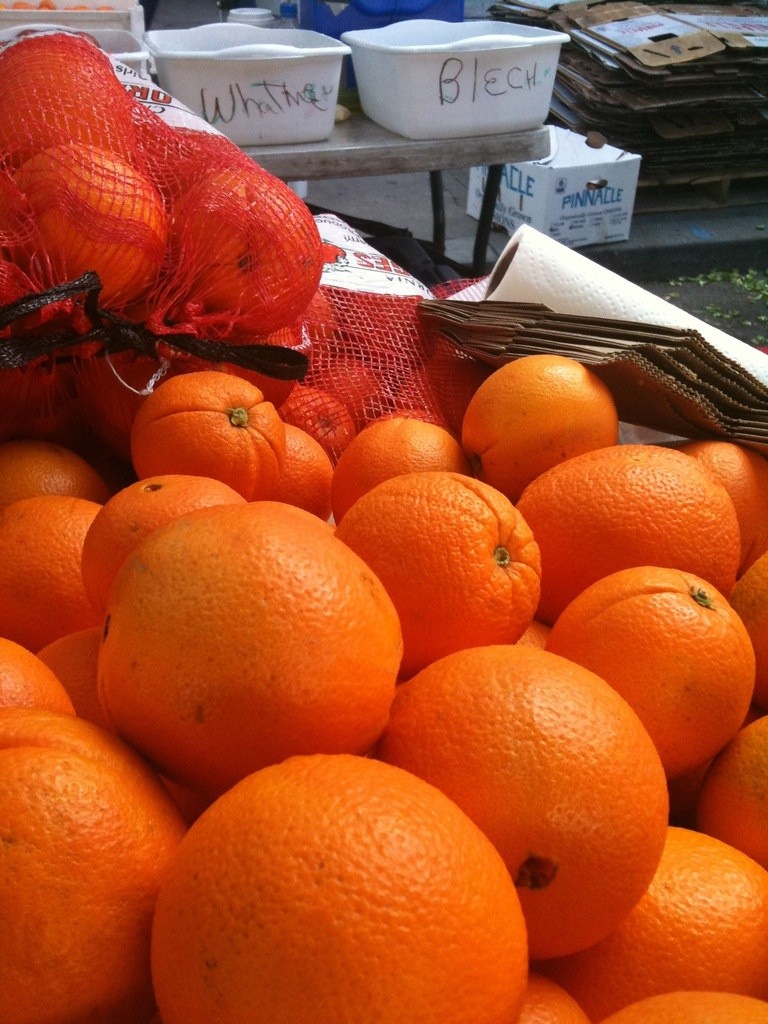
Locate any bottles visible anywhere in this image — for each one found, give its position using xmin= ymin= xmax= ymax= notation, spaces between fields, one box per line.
xmin=226 ymin=7 xmax=276 ymax=29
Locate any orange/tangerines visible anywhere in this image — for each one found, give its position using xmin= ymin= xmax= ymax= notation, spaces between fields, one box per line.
xmin=0 ymin=31 xmax=768 ymax=1024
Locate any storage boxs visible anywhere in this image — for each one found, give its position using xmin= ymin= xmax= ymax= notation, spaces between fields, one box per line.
xmin=467 ymin=123 xmax=642 ymax=251
xmin=0 ymin=0 xmax=352 ymax=148
xmin=342 ymin=19 xmax=571 ymax=139
